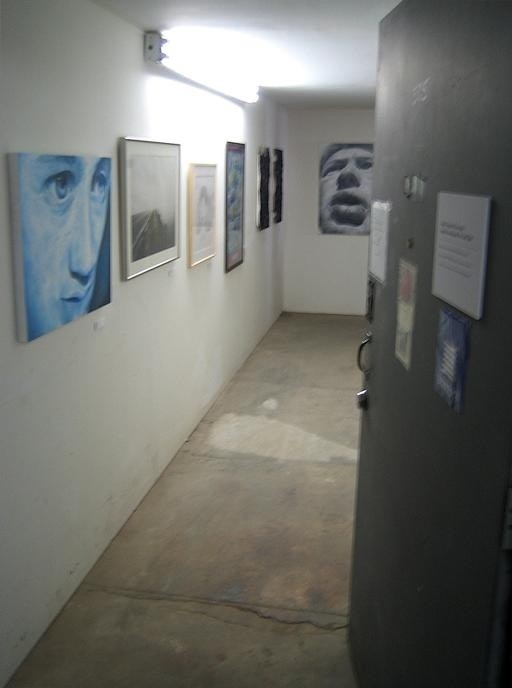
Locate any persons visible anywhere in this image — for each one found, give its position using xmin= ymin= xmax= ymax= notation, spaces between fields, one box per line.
xmin=19 ymin=151 xmax=110 ymax=341
xmin=317 ymin=143 xmax=373 ymax=235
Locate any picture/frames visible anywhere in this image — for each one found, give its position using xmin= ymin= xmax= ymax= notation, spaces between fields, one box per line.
xmin=118 ymin=136 xmax=246 ymax=281
